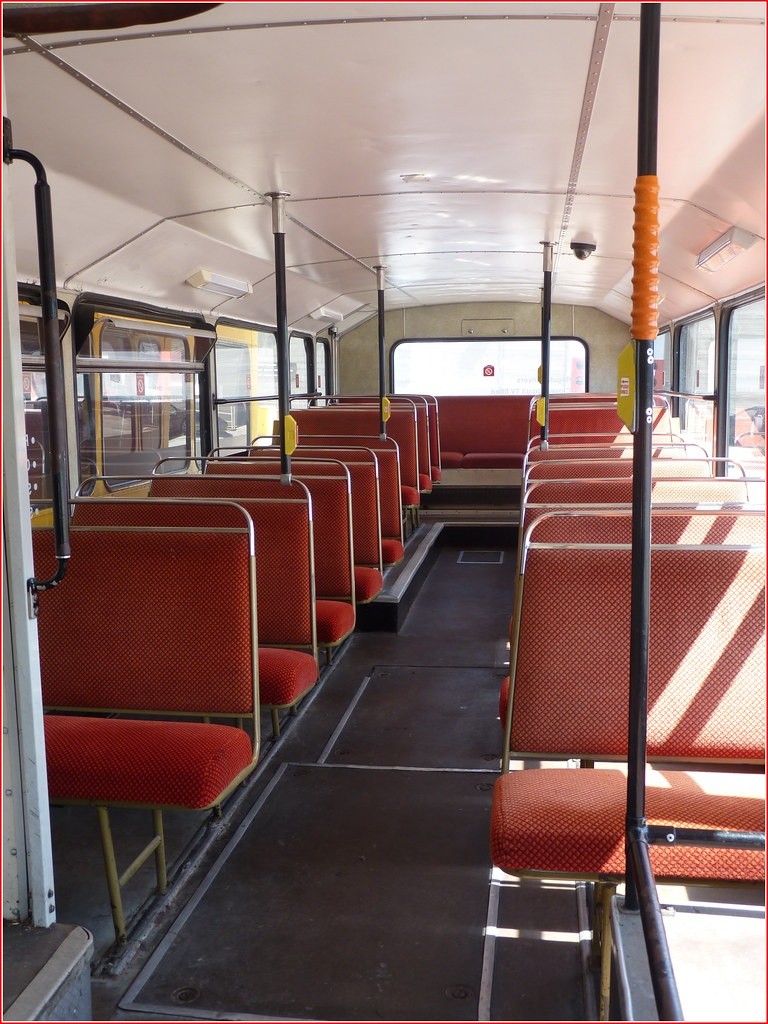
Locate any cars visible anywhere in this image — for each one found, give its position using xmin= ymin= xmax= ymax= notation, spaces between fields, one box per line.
xmin=705 ymin=405 xmax=766 ymax=448
xmin=143 ymin=403 xmax=188 ymax=437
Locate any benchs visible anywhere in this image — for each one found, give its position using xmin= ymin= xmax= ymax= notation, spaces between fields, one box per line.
xmin=335 ymin=394 xmax=672 ymax=473
xmin=23 ymin=390 xmax=250 ymax=514
xmin=70 ymin=474 xmax=319 ymax=821
xmin=508 ymin=456 xmax=749 ymax=650
xmin=30 ymin=496 xmax=263 ymax=948
xmin=150 ymin=455 xmax=358 ymax=719
xmin=203 ymin=445 xmax=385 ymax=668
xmin=246 ymin=395 xmax=442 ymax=569
xmin=683 ymin=396 xmax=766 ymax=478
xmin=490 ymin=508 xmax=766 ymax=1024
xmin=520 ymin=393 xmax=714 ymax=489
xmin=498 ymin=477 xmax=768 ymax=769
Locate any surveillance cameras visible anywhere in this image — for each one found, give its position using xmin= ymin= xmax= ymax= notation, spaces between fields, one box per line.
xmin=570 ymin=240 xmax=597 ymax=260
